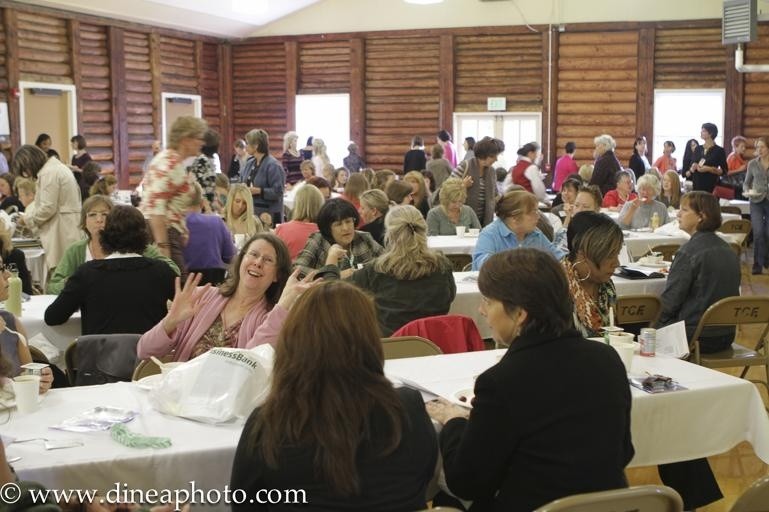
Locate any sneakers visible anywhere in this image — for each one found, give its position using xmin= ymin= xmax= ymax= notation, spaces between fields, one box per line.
xmin=751 ymin=263 xmax=762 ymax=273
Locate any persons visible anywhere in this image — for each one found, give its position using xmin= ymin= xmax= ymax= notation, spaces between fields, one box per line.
xmin=48 ymin=194 xmax=182 ymax=297
xmin=424 ymin=249 xmax=632 ymax=512
xmin=232 ymin=280 xmax=439 ymax=512
xmin=1 ymin=130 xmax=119 ymax=276
xmin=46 ymin=205 xmax=177 ymax=335
xmin=130 ymin=115 xmax=769 ymax=376
xmin=1 ymin=211 xmax=35 ymax=294
xmin=1 ymin=256 xmax=55 ymax=395
xmin=1 ymin=440 xmax=64 ymax=512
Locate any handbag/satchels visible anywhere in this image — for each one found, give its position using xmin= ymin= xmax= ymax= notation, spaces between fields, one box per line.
xmin=717 ymin=170 xmax=745 ymax=188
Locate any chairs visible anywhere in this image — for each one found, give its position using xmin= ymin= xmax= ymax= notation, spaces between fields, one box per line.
xmin=0 ymin=183 xmax=768 ymax=512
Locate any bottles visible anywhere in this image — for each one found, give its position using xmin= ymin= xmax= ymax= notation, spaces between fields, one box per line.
xmin=651 ymin=212 xmax=660 ymax=229
xmin=5 ymin=268 xmax=23 ymax=319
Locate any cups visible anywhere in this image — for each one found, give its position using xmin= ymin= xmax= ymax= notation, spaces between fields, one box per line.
xmin=648 ymin=254 xmax=663 ymax=264
xmin=468 ymin=228 xmax=480 ymax=235
xmin=10 ymin=375 xmax=41 ymax=416
xmin=20 ymin=363 xmax=49 ymax=378
xmin=749 ymin=189 xmax=757 ymax=194
xmin=456 ymin=225 xmax=466 ymax=238
xmin=601 ymin=326 xmax=625 ymax=343
xmin=607 ymin=332 xmax=636 ymax=372
xmin=234 ymin=234 xmax=245 ymax=246
xmin=160 ymin=362 xmax=182 ymax=378
xmin=119 ymin=190 xmax=132 ymax=203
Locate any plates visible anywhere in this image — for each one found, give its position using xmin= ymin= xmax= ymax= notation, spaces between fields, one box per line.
xmin=638 ymin=262 xmax=667 ymax=268
xmin=464 ymin=233 xmax=480 ymax=238
xmin=742 ymin=192 xmax=761 ymax=198
xmin=135 ymin=372 xmax=161 ymax=392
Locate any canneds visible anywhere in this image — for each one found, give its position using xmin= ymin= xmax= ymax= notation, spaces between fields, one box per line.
xmin=640 ymin=328 xmax=656 ymax=356
